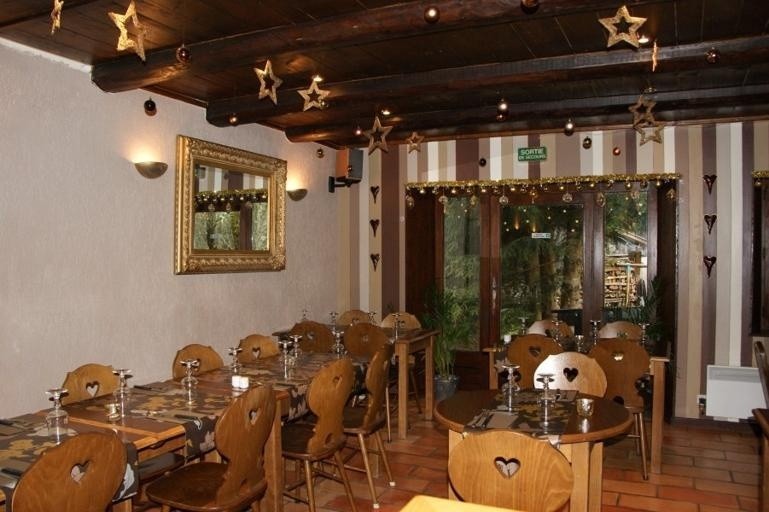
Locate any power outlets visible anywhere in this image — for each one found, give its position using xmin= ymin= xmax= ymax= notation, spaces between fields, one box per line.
xmin=696 ymin=394 xmax=707 ymax=406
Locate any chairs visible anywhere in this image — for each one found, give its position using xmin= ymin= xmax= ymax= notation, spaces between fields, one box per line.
xmin=280 ymin=359 xmax=358 ymax=512
xmin=289 ymin=319 xmax=338 ymax=357
xmin=237 ymin=334 xmax=281 ymax=366
xmin=506 ymin=333 xmax=565 ymax=389
xmin=145 ymin=385 xmax=277 ymax=512
xmin=343 ymin=322 xmax=412 ymax=443
xmin=587 ymin=338 xmax=650 ymax=481
xmin=400 ymin=495 xmax=530 ymax=512
xmin=526 ymin=318 xmax=575 ymax=346
xmin=302 ymin=343 xmax=396 ymax=509
xmin=173 ymin=343 xmax=225 ymax=381
xmin=533 ymin=352 xmax=607 ymax=398
xmin=59 ymin=363 xmax=129 ymax=407
xmin=381 ymin=311 xmax=424 ymax=415
xmin=449 ymin=431 xmax=573 ymax=512
xmin=597 ymin=320 xmax=650 ymax=456
xmin=753 ymin=341 xmax=769 ymax=409
xmin=10 ymin=432 xmax=128 ymax=512
xmin=336 ymin=308 xmax=373 ymax=335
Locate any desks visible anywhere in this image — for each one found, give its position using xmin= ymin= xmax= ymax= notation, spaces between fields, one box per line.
xmin=273 ymin=322 xmax=441 ymax=441
xmin=236 ymin=349 xmax=398 ymax=480
xmin=166 ymin=359 xmax=368 ymax=419
xmin=1 ymin=412 xmax=158 ymax=512
xmin=38 ymin=380 xmax=291 ymax=512
xmin=482 ymin=333 xmax=672 ymax=475
xmin=432 ymin=388 xmax=635 ymax=512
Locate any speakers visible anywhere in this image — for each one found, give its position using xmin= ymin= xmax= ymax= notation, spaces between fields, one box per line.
xmin=336 ymin=149 xmax=362 ymax=181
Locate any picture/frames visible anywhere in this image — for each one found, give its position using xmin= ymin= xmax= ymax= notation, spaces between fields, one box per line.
xmin=173 ymin=134 xmax=287 ymax=274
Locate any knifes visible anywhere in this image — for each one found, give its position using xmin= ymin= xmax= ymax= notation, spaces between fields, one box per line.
xmin=0 ymin=418 xmax=27 ymax=430
xmin=155 ymin=414 xmax=198 ymax=421
xmin=481 ymin=414 xmax=494 ymax=429
xmin=555 ymin=388 xmax=561 ymax=401
xmin=135 ymin=385 xmax=162 ymax=392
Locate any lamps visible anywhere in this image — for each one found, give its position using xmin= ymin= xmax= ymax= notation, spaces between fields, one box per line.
xmin=497 ymin=96 xmax=509 ymax=115
xmin=228 ymin=112 xmax=239 ymax=126
xmin=425 ymin=4 xmax=440 ymax=25
xmin=135 ymin=161 xmax=168 ymax=179
xmin=636 ymin=32 xmax=652 ymax=47
xmin=520 ymin=0 xmax=541 ymax=17
xmin=378 ymin=103 xmax=395 ymax=118
xmin=564 ymin=117 xmax=574 ymax=137
xmin=286 ymin=187 xmax=308 ymax=202
xmin=143 ymin=98 xmax=157 ymax=117
xmin=352 ymin=126 xmax=363 ymax=139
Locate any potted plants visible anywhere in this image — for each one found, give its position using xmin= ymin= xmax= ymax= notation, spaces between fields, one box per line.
xmin=600 ymin=275 xmax=673 ymax=358
xmin=421 ymin=284 xmax=480 ymax=400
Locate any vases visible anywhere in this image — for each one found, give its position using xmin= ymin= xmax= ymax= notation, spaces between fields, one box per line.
xmin=434 ymin=374 xmax=460 ymax=401
